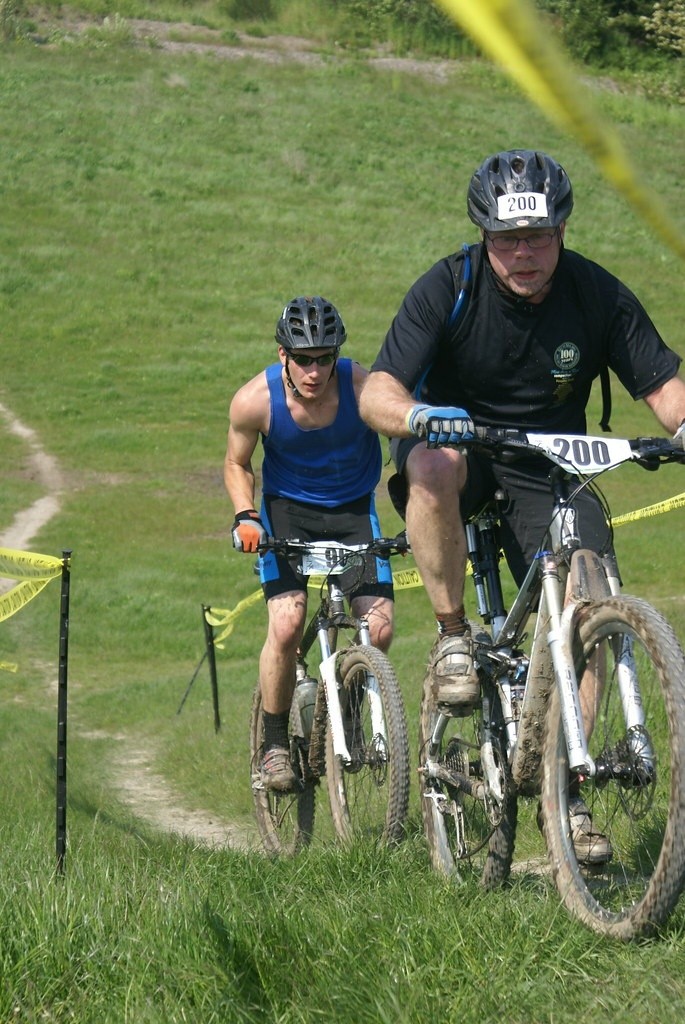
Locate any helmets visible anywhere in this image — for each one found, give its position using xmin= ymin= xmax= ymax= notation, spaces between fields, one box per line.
xmin=465 ymin=149 xmax=575 ymax=234
xmin=274 ymin=297 xmax=351 ymax=351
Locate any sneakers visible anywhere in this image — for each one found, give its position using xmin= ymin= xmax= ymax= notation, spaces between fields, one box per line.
xmin=258 ymin=743 xmax=301 ymax=795
xmin=429 ymin=628 xmax=480 ymax=706
xmin=536 ymin=791 xmax=614 ymax=862
xmin=342 ymin=706 xmax=367 ymax=774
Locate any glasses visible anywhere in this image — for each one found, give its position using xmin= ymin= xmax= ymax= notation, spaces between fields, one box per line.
xmin=485 ymin=229 xmax=559 ymax=251
xmin=287 ymin=349 xmax=337 ymax=367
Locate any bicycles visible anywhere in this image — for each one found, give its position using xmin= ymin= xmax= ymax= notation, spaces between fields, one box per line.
xmin=415 ymin=423 xmax=684 ymax=947
xmin=233 ymin=526 xmax=415 ymax=863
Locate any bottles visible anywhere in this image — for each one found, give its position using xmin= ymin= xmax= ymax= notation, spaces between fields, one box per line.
xmin=295 ymin=675 xmax=318 ymax=745
xmin=509 ymin=659 xmax=529 ymax=736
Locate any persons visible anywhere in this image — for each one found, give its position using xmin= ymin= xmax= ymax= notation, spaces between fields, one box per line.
xmin=358 ymin=137 xmax=683 ymax=862
xmin=223 ymin=297 xmax=399 ymax=795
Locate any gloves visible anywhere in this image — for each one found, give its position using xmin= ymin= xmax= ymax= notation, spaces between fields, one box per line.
xmin=672 ymin=418 xmax=685 ymax=452
xmin=229 ymin=508 xmax=269 ymax=556
xmin=404 ymin=404 xmax=476 ymax=456
